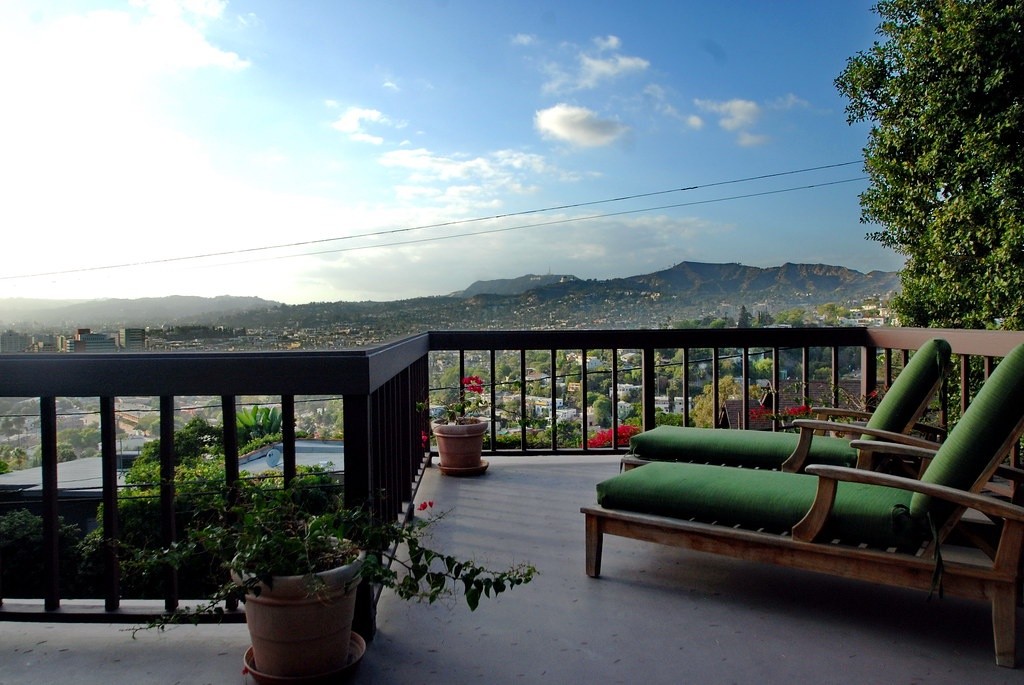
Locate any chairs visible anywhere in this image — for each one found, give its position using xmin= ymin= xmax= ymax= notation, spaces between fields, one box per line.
xmin=580 ymin=343 xmax=1023 ymax=668
xmin=619 ymin=339 xmax=952 ymax=480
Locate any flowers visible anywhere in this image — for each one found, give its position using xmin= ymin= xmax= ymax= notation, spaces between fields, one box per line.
xmin=752 ymin=367 xmax=879 ymax=433
xmin=417 ymin=377 xmax=486 ymax=447
xmin=123 ymin=484 xmax=535 ymax=643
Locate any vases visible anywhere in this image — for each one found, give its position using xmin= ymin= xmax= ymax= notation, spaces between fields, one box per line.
xmin=828 ymin=421 xmax=867 ymax=439
xmin=433 ymin=419 xmax=488 ymax=466
xmin=230 ymin=536 xmax=365 ymax=676
